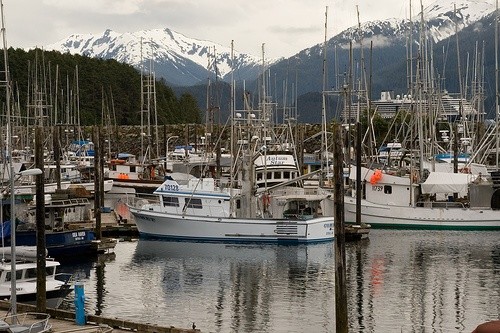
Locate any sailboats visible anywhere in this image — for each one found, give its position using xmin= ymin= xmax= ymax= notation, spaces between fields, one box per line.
xmin=0 ymin=0 xmax=500 ymax=333
